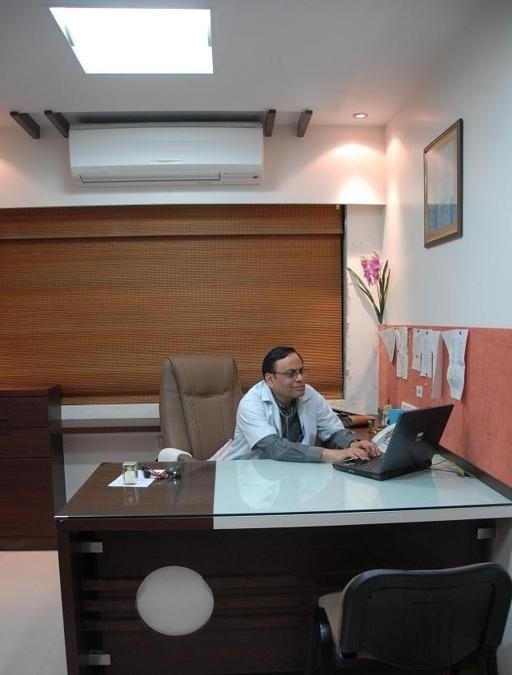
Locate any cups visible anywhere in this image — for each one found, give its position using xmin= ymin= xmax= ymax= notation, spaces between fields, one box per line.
xmin=121 ymin=460 xmax=138 ymax=485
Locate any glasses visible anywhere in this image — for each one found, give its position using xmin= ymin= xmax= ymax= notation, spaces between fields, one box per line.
xmin=272 ymin=367 xmax=308 ymax=379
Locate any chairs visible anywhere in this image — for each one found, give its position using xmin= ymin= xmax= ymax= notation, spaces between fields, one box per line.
xmin=158 ymin=354 xmax=243 ymax=462
xmin=304 ymin=562 xmax=512 ymax=675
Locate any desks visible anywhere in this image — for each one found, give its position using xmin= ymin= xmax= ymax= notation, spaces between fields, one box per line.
xmin=53 ymin=461 xmax=512 ymax=675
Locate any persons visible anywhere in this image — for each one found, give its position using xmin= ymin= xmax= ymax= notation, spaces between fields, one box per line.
xmin=219 ymin=344 xmax=382 ymax=463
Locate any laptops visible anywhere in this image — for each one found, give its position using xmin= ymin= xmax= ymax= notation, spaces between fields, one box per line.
xmin=332 ymin=403 xmax=454 ymax=482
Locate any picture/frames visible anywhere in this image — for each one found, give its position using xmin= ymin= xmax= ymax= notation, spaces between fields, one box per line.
xmin=424 ymin=118 xmax=463 ymax=249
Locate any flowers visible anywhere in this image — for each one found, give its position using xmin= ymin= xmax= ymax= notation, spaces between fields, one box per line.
xmin=347 ymin=250 xmax=390 ymax=325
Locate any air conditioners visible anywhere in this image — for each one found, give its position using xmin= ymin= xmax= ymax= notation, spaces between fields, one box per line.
xmin=69 ymin=123 xmax=264 ymax=185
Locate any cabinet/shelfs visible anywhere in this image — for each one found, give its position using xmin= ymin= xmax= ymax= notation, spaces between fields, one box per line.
xmin=0 ymin=384 xmax=66 ymax=551
